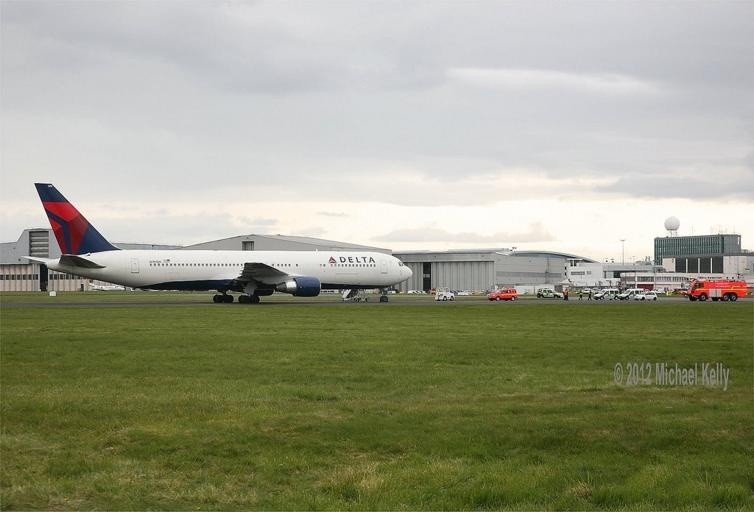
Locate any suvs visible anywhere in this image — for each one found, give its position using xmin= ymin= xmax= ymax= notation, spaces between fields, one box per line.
xmin=487 ymin=288 xmax=518 ymax=301
xmin=537 ymin=288 xmax=563 ymax=298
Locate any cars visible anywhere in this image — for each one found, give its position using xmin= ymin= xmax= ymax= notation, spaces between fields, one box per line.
xmin=636 ymin=292 xmax=658 ymax=301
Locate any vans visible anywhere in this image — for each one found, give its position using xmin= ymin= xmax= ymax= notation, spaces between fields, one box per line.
xmin=619 ymin=289 xmax=643 ymax=301
xmin=595 ymin=289 xmax=619 ymax=300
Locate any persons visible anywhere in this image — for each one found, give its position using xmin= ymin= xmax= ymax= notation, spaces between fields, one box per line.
xmin=577 ymin=289 xmax=584 ymax=300
xmin=587 ymin=289 xmax=593 ymax=300
xmin=562 ymin=288 xmax=570 ymax=300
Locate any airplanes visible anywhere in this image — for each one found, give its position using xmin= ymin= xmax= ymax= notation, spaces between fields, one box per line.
xmin=89 ymin=283 xmax=126 ymax=293
xmin=21 ymin=180 xmax=415 ymax=304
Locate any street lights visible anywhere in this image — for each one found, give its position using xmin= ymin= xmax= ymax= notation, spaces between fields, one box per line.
xmin=621 ymin=240 xmax=626 ymax=266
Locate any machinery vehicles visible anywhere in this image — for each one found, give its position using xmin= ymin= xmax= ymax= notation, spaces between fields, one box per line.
xmin=434 ymin=287 xmax=456 ymax=302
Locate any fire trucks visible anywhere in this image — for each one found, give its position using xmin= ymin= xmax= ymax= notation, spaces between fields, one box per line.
xmin=687 ymin=279 xmax=748 ymax=302
